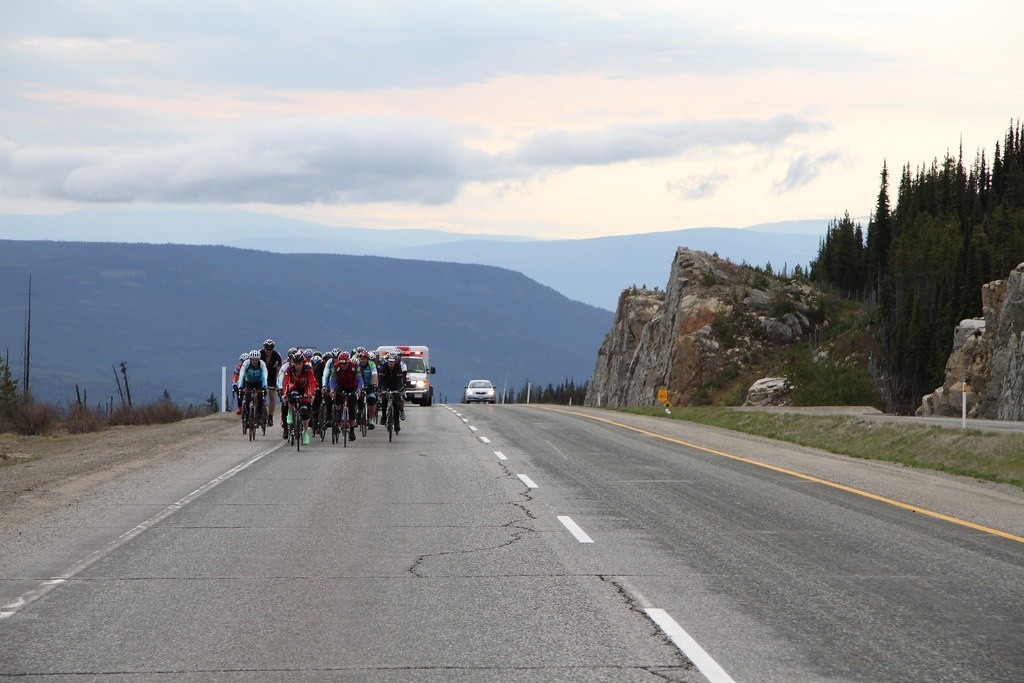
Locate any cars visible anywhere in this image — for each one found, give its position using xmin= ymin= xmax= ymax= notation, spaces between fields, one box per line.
xmin=464 ymin=380 xmax=497 ymax=403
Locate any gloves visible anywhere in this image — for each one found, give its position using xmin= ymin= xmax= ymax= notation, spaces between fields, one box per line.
xmin=262 ymin=387 xmax=267 ymax=397
xmin=237 ymin=387 xmax=242 ymax=394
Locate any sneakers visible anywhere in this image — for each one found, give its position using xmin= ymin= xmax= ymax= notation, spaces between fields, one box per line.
xmin=236 ymin=401 xmax=405 ymax=445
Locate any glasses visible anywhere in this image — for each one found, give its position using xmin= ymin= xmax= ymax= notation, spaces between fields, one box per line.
xmin=265 ymin=348 xmax=273 ymax=350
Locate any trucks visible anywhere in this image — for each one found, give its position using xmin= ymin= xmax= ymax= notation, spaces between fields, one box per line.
xmin=377 ymin=345 xmax=436 ymax=406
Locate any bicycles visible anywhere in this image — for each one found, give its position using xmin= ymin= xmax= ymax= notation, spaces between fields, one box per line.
xmin=231 ymin=386 xmax=407 ymax=451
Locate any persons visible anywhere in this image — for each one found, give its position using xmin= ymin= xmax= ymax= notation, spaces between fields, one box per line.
xmin=281 ymin=352 xmax=315 ymax=445
xmin=259 ymin=339 xmax=282 ymax=426
xmin=232 ymin=353 xmax=249 ymax=415
xmin=412 ymin=362 xmax=422 ymax=370
xmin=276 ymin=348 xmax=407 ymax=441
xmin=238 ymin=350 xmax=267 ymax=419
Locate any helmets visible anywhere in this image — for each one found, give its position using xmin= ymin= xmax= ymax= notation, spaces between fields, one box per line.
xmin=288 ymin=347 xmax=405 ymax=365
xmin=248 ymin=350 xmax=261 ymax=359
xmin=239 ymin=353 xmax=248 ymax=360
xmin=263 ymin=339 xmax=275 ymax=346
